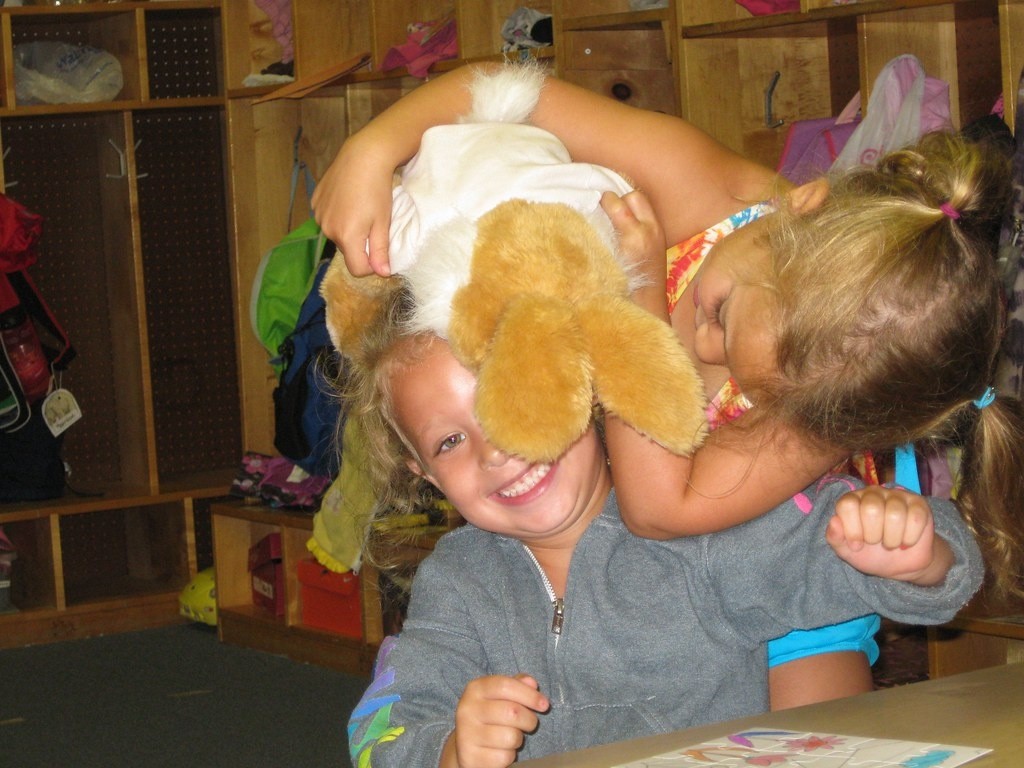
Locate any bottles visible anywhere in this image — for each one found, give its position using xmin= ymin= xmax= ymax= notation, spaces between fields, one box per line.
xmin=0 ymin=308 xmax=51 ymax=399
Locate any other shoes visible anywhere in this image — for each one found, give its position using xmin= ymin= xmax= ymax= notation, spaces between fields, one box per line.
xmin=225 ymin=454 xmax=272 ymax=505
xmin=259 ymin=458 xmax=329 ymax=510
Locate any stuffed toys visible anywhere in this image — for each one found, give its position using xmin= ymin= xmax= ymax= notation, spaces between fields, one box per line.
xmin=317 ymin=69 xmax=709 ymax=464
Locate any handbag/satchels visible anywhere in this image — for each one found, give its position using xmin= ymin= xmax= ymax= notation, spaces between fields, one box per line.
xmin=994 ymin=72 xmax=1023 ymax=405
xmin=249 ymin=217 xmax=328 ymax=380
xmin=14 ymin=41 xmax=125 ymax=106
xmin=0 ymin=388 xmax=66 ymax=501
xmin=828 ymin=54 xmax=926 ymax=177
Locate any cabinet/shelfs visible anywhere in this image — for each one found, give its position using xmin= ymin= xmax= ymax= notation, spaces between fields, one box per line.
xmin=1 ymin=0 xmax=1023 ymax=679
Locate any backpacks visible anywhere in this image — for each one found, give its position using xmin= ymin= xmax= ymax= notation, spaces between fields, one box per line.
xmin=272 ymin=240 xmax=349 ymax=482
xmin=778 ymin=90 xmax=861 ymax=185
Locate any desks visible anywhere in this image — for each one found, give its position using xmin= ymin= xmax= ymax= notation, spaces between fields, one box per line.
xmin=508 ymin=664 xmax=1024 ymax=768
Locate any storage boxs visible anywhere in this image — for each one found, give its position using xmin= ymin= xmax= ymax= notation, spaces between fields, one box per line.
xmin=297 ymin=560 xmax=361 ymax=637
xmin=248 ymin=533 xmax=284 ymax=618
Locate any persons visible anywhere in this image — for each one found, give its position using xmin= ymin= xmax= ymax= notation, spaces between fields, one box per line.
xmin=309 ymin=61 xmax=1024 ymax=713
xmin=330 ymin=285 xmax=985 ymax=768
